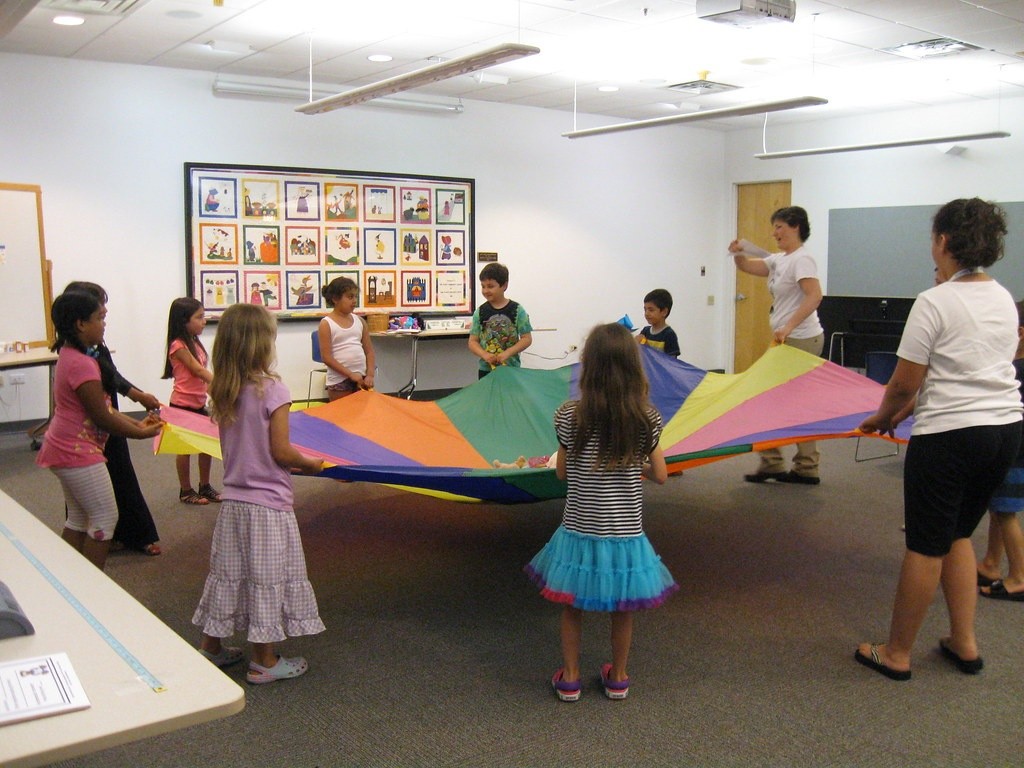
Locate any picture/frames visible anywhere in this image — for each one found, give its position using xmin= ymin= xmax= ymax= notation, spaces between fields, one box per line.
xmin=183 ymin=160 xmax=477 ymax=325
xmin=0 ymin=182 xmax=60 ymax=351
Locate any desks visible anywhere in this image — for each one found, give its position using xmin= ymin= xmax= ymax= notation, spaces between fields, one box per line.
xmin=0 ymin=344 xmax=117 ymax=450
xmin=364 ymin=326 xmax=556 ymax=400
xmin=0 ymin=489 xmax=246 ymax=768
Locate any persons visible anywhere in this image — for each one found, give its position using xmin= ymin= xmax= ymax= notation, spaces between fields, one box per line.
xmin=162 ymin=298 xmax=222 ymax=504
xmin=468 ymin=263 xmax=532 ymax=380
xmin=634 ymin=288 xmax=683 ymax=477
xmin=728 ymin=206 xmax=824 ymax=484
xmin=50 ymin=282 xmax=162 ymax=556
xmin=978 ymin=302 xmax=1024 ymax=601
xmin=319 ymin=277 xmax=375 ymax=401
xmin=35 ymin=292 xmax=165 ymax=571
xmin=856 ymin=200 xmax=1024 ymax=681
xmin=192 ymin=303 xmax=327 ymax=682
xmin=523 ymin=322 xmax=678 ymax=701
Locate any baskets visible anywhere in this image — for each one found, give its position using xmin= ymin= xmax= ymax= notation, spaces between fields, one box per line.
xmin=365 ymin=312 xmax=390 ymax=334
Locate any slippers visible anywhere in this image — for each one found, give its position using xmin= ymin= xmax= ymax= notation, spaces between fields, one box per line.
xmin=939 ymin=635 xmax=983 ymax=675
xmin=973 ymin=568 xmax=1003 ymax=587
xmin=979 ymin=578 xmax=1024 ymax=602
xmin=854 ymin=641 xmax=912 ymax=681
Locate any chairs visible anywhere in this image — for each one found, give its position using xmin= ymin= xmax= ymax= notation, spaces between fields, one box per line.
xmin=855 ymin=351 xmax=902 ymax=462
xmin=307 ymin=331 xmax=377 ymax=407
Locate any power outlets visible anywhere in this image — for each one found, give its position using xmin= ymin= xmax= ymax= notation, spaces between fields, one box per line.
xmin=478 ymin=252 xmax=497 ymax=260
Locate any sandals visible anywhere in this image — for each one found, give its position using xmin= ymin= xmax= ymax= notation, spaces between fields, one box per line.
xmin=245 ymin=653 xmax=310 ymax=684
xmin=107 ymin=540 xmax=127 ymax=552
xmin=599 ymin=663 xmax=631 ymax=700
xmin=138 ymin=541 xmax=162 ymax=555
xmin=197 ymin=643 xmax=245 ymax=666
xmin=178 ymin=487 xmax=210 ymax=505
xmin=197 ymin=482 xmax=223 ymax=502
xmin=550 ymin=668 xmax=582 ymax=702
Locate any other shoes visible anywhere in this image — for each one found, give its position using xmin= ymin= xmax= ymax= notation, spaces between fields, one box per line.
xmin=776 ymin=469 xmax=820 ymax=485
xmin=744 ymin=470 xmax=786 ymax=483
